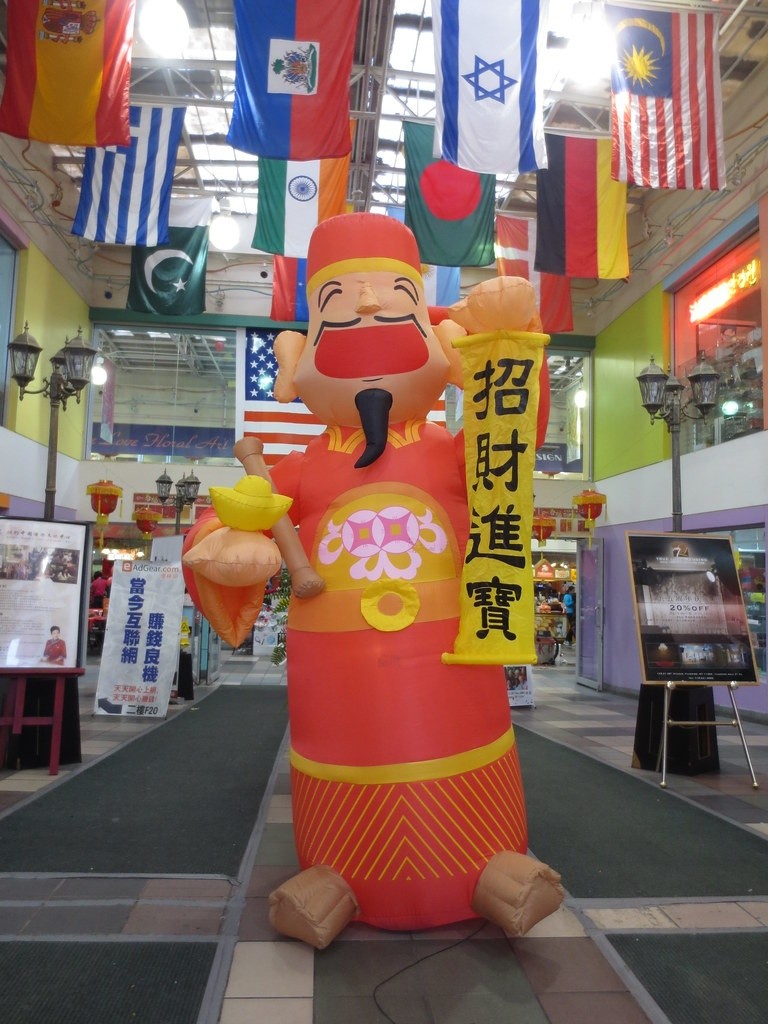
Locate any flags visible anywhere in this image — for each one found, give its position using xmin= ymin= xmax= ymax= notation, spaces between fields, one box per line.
xmin=126 ymin=196 xmax=214 ymax=316
xmin=70 ymin=105 xmax=187 ymax=248
xmin=534 ymin=127 xmax=629 ymax=279
xmin=401 ymin=121 xmax=495 ymax=268
xmin=225 ymin=0 xmax=361 ymax=161
xmin=431 ymin=0 xmax=550 ymax=174
xmin=269 ymin=203 xmax=355 ymax=321
xmin=495 ymin=211 xmax=574 ymax=334
xmin=387 ymin=205 xmax=460 ymax=307
xmin=0 ymin=0 xmax=136 ymax=146
xmin=250 ymin=119 xmax=358 ymax=259
xmin=605 ymin=5 xmax=726 ymax=190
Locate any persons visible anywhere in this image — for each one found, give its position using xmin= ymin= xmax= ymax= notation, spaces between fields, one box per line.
xmin=90 ymin=572 xmax=112 ymax=627
xmin=720 ymin=325 xmax=737 ymax=342
xmin=40 ymin=626 xmax=67 ymax=666
xmin=181 ymin=213 xmax=564 ymax=948
xmin=562 ymin=586 xmax=577 ymax=646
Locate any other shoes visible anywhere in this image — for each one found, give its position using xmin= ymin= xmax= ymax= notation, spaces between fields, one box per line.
xmin=563 ymin=641 xmax=569 ymax=646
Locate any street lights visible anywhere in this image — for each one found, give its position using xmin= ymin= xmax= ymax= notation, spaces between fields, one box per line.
xmin=1 ymin=320 xmax=98 ymax=767
xmin=631 ymin=350 xmax=719 ymax=775
xmin=155 ymin=467 xmax=201 ymax=535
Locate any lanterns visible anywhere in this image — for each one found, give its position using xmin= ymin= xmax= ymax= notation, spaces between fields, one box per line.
xmin=572 ymin=490 xmax=607 ymax=550
xmin=532 ymin=517 xmax=555 ymax=562
xmin=87 ymin=479 xmax=123 ymax=550
xmin=132 ymin=508 xmax=162 ymax=549
